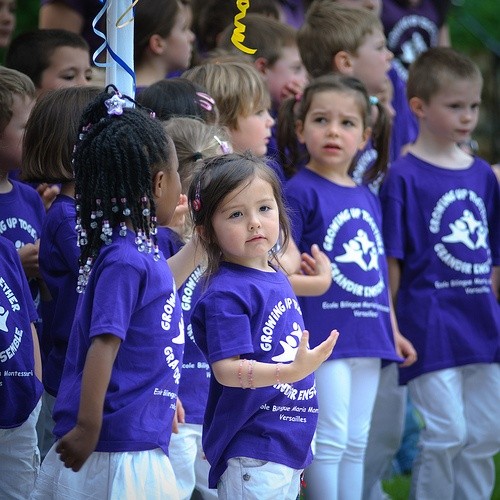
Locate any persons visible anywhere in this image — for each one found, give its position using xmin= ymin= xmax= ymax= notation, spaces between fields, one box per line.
xmin=30 ymin=83 xmax=185 ymax=500
xmin=281 ymin=74 xmax=417 ymax=500
xmin=0 ymin=0 xmax=455 ymax=500
xmin=378 ymin=46 xmax=500 ymax=500
xmin=187 ymin=149 xmax=340 ymax=500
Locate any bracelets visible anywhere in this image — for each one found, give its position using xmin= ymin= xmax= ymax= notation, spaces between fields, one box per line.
xmin=237 ymin=359 xmax=281 ymax=390
